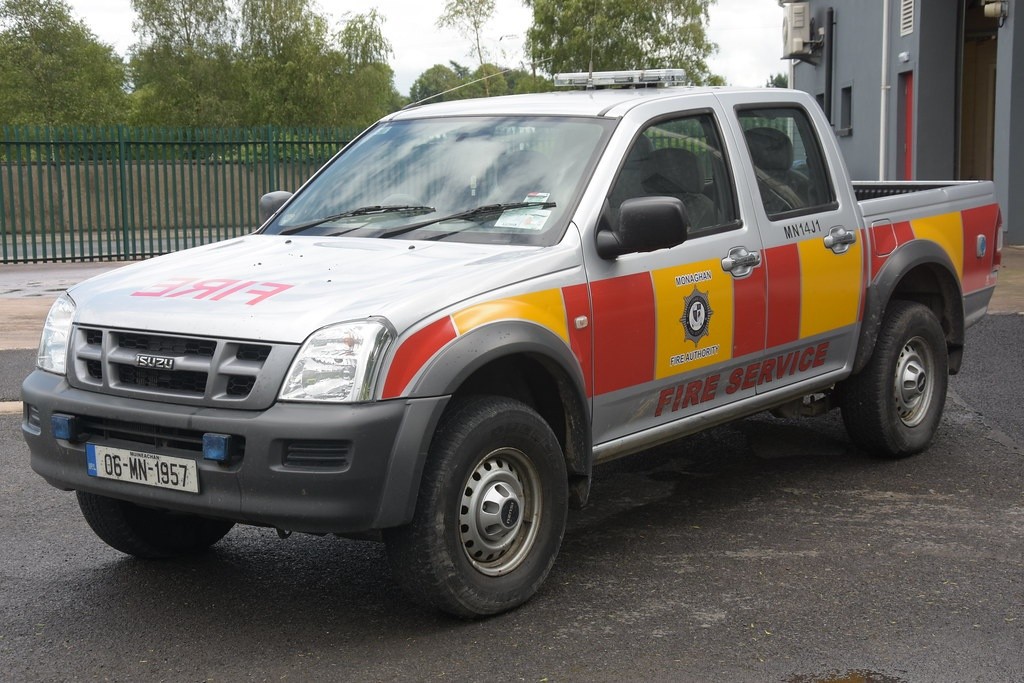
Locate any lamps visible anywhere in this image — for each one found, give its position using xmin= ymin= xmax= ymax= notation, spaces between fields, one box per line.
xmin=984 ymin=2 xmax=1009 ymax=17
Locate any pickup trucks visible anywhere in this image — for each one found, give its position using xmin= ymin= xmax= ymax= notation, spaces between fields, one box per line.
xmin=20 ymin=67 xmax=1005 ymax=618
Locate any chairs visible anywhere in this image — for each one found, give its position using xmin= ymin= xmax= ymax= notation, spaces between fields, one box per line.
xmin=744 ymin=127 xmax=817 ymax=215
xmin=640 ymin=148 xmax=724 ymax=232
xmin=608 ymin=134 xmax=652 ymax=207
xmin=497 ymin=150 xmax=552 ymax=198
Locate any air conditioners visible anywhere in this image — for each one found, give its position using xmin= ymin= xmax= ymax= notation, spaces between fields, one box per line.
xmin=782 ymin=2 xmax=810 ymax=59
